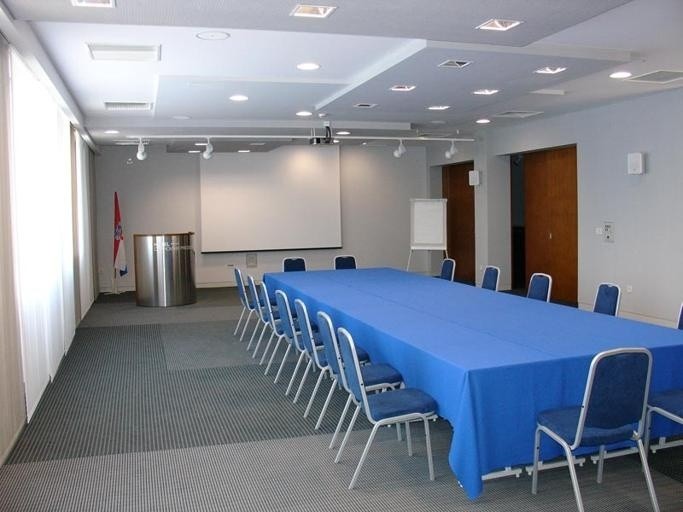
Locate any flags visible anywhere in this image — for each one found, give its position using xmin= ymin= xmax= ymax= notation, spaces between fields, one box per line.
xmin=113 ymin=191 xmax=128 ymax=276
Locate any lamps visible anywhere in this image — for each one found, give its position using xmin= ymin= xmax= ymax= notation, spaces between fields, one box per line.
xmin=444 ymin=140 xmax=458 ymax=160
xmin=392 ymin=140 xmax=407 ymax=157
xmin=136 ymin=138 xmax=147 ymax=160
xmin=202 ymin=137 xmax=213 ymax=159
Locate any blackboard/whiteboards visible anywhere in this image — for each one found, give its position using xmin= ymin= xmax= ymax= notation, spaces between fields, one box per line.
xmin=409 ymin=199 xmax=448 ymax=251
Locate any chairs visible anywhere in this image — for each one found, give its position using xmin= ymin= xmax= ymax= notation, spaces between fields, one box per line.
xmin=677 ymin=303 xmax=683 ymax=331
xmin=593 ymin=283 xmax=621 ymax=317
xmin=333 ymin=255 xmax=357 ymax=270
xmin=440 ymin=258 xmax=456 ymax=281
xmin=481 ymin=265 xmax=500 ymax=291
xmin=532 ymin=347 xmax=660 ymax=512
xmin=526 ymin=272 xmax=552 ymax=303
xmin=642 ymin=388 xmax=683 ymax=473
xmin=282 ymin=257 xmax=307 ymax=272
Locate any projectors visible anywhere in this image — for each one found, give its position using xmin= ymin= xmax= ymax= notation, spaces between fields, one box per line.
xmin=310 ymin=137 xmax=334 ymax=146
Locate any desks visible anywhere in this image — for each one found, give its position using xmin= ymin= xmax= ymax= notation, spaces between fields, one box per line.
xmin=260 ymin=268 xmax=683 ymax=501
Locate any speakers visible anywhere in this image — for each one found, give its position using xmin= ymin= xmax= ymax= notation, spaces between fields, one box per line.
xmin=627 ymin=152 xmax=646 ymax=175
xmin=469 ymin=170 xmax=480 ymax=186
